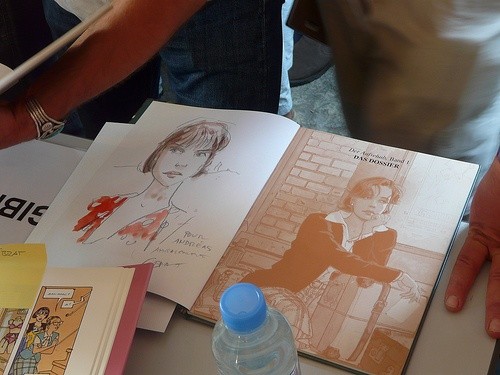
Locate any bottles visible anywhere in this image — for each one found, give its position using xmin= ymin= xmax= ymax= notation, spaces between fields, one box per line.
xmin=209 ymin=283 xmax=301 ymax=375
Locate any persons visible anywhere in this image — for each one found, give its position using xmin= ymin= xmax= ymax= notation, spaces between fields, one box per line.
xmin=0 ymin=0 xmax=334 ymax=150
xmin=324 ymin=0 xmax=500 ymax=339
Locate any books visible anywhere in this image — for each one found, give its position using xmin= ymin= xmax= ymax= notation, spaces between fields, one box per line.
xmin=42 ymin=100 xmax=480 ymax=375
xmin=0 ymin=262 xmax=153 ymax=375
xmin=0 ymin=6 xmax=114 ymax=97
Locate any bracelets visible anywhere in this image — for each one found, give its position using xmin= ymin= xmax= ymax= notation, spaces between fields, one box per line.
xmin=24 ymin=96 xmax=65 ymax=141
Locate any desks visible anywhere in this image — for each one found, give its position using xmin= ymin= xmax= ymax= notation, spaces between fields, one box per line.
xmin=0 ymin=133 xmax=497 ymax=375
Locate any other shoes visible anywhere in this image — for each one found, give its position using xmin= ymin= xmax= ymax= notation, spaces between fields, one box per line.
xmin=287 ymin=36 xmax=334 ymax=85
